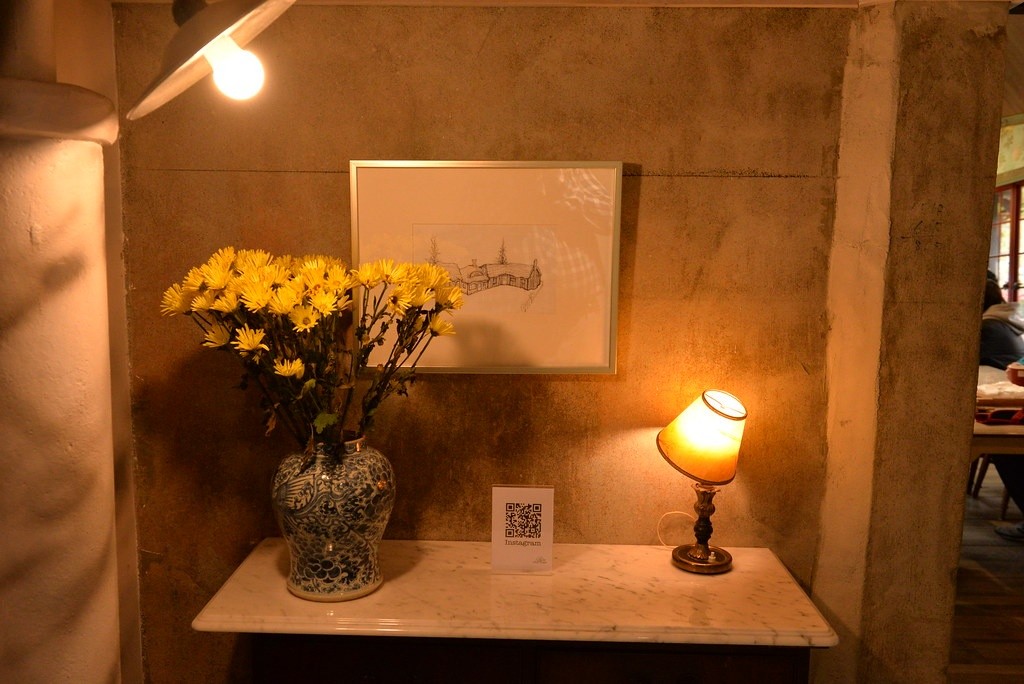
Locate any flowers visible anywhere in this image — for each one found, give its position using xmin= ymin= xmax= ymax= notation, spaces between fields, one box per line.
xmin=159 ymin=245 xmax=465 ymax=456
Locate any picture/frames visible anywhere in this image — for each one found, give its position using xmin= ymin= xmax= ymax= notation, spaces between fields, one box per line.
xmin=349 ymin=159 xmax=624 ymax=375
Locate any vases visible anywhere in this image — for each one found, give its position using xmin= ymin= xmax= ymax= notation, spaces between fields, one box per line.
xmin=269 ymin=431 xmax=398 ymax=604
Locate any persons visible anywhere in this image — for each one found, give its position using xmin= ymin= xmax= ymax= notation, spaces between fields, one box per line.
xmin=980 ymin=270 xmax=1024 ymax=371
xmin=991 ymin=356 xmax=1024 ymax=542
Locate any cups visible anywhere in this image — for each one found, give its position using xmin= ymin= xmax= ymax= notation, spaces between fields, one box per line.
xmin=1005 ymin=362 xmax=1024 ymax=387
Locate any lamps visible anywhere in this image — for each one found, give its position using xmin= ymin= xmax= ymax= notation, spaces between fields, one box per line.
xmin=126 ymin=0 xmax=298 ymax=122
xmin=655 ymin=389 xmax=748 ymax=575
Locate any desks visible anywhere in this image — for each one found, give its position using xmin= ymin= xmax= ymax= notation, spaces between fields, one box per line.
xmin=192 ymin=536 xmax=840 ymax=684
xmin=967 ymin=419 xmax=1024 ymax=498
